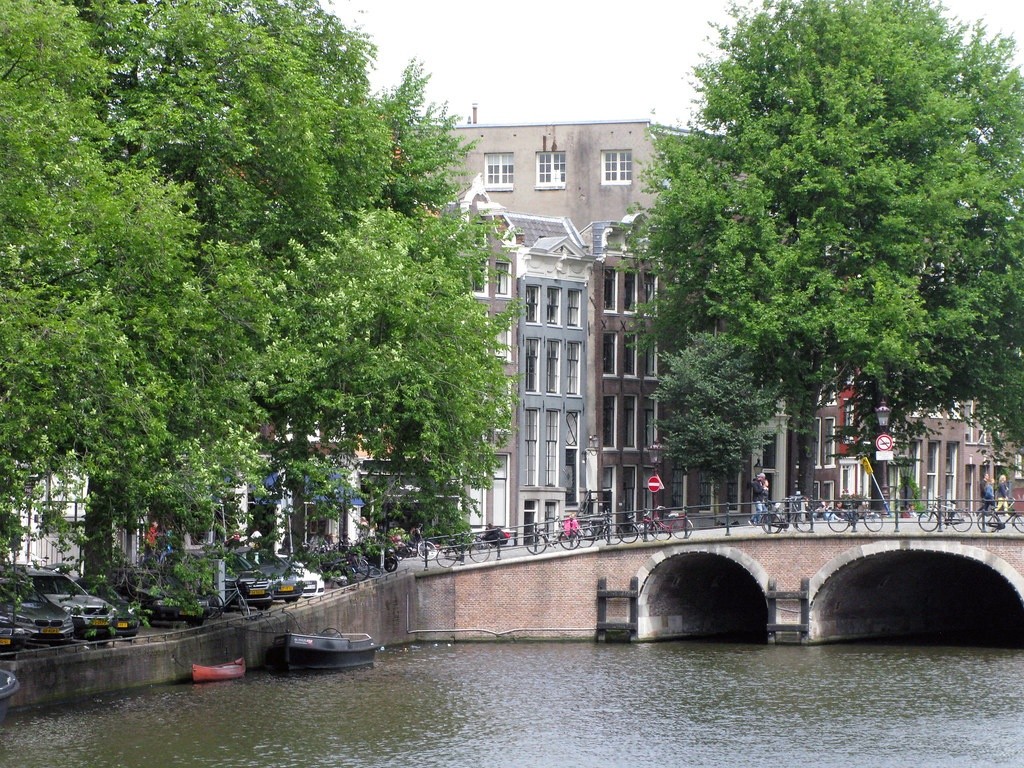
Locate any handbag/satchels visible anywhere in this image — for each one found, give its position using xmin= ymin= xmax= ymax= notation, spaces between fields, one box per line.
xmin=502 ymin=531 xmax=510 ymax=538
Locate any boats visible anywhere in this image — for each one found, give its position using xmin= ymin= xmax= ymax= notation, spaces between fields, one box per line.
xmin=192 ymin=656 xmax=247 ymax=684
xmin=273 ymin=627 xmax=377 ymax=671
xmin=0 ymin=668 xmax=20 ymax=723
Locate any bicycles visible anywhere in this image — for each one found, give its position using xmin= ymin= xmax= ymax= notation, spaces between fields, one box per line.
xmin=761 ymin=496 xmax=812 ymax=533
xmin=828 ymin=501 xmax=882 ymax=532
xmin=390 ymin=524 xmax=438 ymax=561
xmin=525 ymin=506 xmax=693 ymax=554
xmin=436 ymin=529 xmax=490 ymax=569
xmin=201 ymin=574 xmax=251 ymax=620
xmin=977 ymin=498 xmax=1024 ymax=533
xmin=333 ymin=543 xmax=383 ymax=586
xmin=917 ymin=494 xmax=974 ymax=532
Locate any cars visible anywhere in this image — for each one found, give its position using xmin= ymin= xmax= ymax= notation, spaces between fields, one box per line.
xmin=0 ymin=552 xmax=325 ymax=661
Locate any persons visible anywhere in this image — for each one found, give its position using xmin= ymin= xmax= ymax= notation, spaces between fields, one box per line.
xmin=974 ymin=473 xmax=1011 ymax=517
xmin=748 ymin=473 xmax=770 ymax=526
xmin=800 ymin=496 xmax=917 ymax=522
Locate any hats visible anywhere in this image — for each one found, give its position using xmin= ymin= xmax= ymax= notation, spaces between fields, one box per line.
xmin=757 ymin=473 xmax=765 ymax=477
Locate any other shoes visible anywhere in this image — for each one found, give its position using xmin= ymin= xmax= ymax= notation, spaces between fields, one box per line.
xmin=748 ymin=520 xmax=753 ymax=527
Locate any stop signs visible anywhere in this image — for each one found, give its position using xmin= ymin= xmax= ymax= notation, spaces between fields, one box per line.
xmin=648 ymin=477 xmax=660 ymax=494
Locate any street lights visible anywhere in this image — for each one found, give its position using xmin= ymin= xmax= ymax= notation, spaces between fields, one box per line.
xmin=647 ymin=439 xmax=663 ymax=530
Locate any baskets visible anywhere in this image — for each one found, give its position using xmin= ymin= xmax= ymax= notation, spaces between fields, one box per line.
xmin=237 ymin=582 xmax=250 ymax=596
